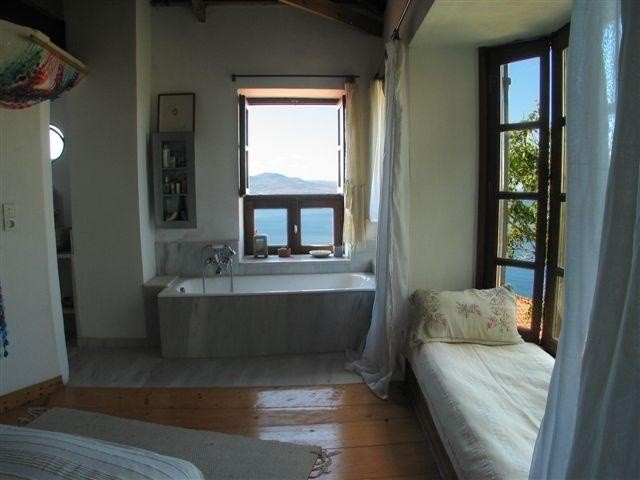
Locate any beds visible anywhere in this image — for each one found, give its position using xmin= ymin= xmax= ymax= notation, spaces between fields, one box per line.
xmin=401 ymin=326 xmax=558 ymax=480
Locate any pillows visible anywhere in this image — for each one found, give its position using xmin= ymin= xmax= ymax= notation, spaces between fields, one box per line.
xmin=407 ymin=282 xmax=526 ymax=350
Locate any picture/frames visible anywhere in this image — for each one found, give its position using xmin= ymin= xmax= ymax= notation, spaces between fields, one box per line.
xmin=155 ymin=92 xmax=196 ymax=134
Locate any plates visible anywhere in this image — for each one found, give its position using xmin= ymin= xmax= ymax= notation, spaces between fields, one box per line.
xmin=310 ymin=249 xmax=331 ymax=257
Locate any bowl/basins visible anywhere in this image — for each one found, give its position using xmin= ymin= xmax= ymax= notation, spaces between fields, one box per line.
xmin=278 ymin=248 xmax=292 ymax=257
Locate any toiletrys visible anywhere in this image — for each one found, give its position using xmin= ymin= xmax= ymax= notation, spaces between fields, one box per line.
xmin=162 ymin=145 xmax=187 ymax=222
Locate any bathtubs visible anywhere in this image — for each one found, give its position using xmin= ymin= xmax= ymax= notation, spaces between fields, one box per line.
xmin=155 ymin=270 xmax=377 ymax=359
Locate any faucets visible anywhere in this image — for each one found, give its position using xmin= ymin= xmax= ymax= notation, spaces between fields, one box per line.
xmin=215 ymin=266 xmax=222 ymax=276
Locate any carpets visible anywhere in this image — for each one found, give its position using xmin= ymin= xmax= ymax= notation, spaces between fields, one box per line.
xmin=12 ymin=405 xmax=342 ymax=479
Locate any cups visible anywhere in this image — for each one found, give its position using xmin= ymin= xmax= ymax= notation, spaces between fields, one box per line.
xmin=168 ymin=156 xmax=177 ymax=168
xmin=333 ymin=245 xmax=344 ymax=257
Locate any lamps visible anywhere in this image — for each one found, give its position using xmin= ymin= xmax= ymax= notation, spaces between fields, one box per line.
xmin=48 ymin=122 xmax=66 ymax=164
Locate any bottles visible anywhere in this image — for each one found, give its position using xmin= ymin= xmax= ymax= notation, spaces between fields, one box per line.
xmin=178 ymin=196 xmax=187 ymax=220
xmin=164 ymin=173 xmax=183 ymax=194
xmin=162 ymin=144 xmax=170 ymax=167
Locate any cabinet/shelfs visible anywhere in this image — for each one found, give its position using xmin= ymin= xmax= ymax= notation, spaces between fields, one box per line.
xmin=151 ymin=132 xmax=198 ymax=231
